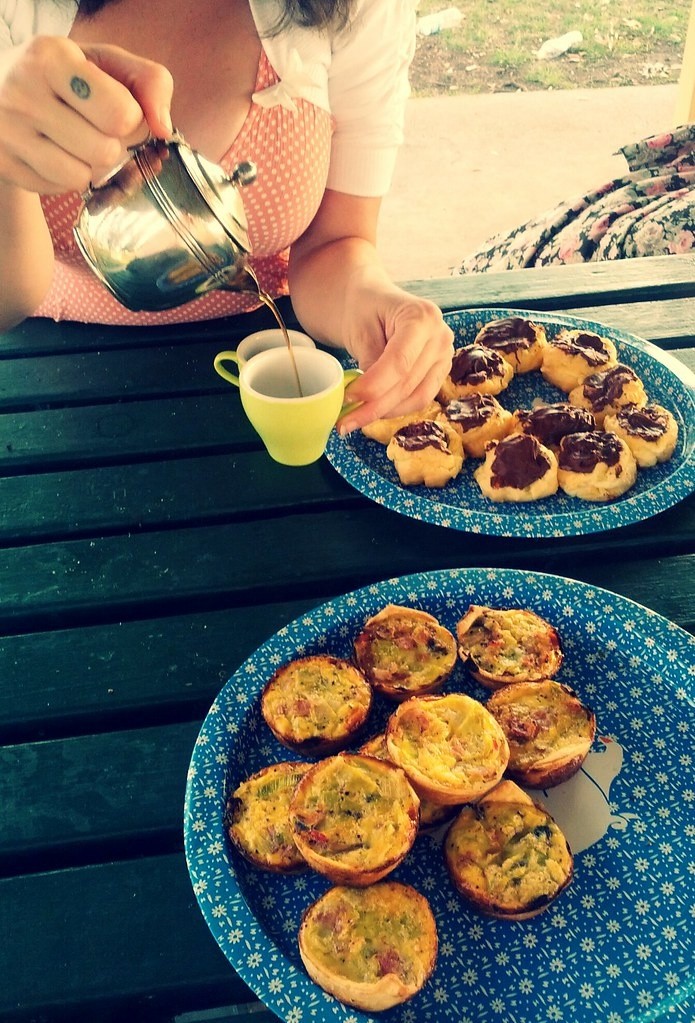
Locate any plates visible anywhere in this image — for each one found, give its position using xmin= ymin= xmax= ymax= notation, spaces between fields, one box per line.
xmin=184 ymin=567 xmax=695 ymax=1023
xmin=324 ymin=309 xmax=695 ymax=538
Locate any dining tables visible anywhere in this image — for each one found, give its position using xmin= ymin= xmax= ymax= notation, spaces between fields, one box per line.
xmin=1 ymin=252 xmax=695 ymax=1023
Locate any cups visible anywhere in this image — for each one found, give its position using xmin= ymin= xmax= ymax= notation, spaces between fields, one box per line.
xmin=214 ymin=329 xmax=317 ymax=388
xmin=238 ymin=345 xmax=365 ymax=467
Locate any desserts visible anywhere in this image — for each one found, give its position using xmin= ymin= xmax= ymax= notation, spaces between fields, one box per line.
xmin=363 ymin=316 xmax=679 ymax=502
xmin=230 ymin=602 xmax=599 ymax=1012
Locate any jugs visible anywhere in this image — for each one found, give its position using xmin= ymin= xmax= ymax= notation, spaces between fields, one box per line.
xmin=74 ymin=126 xmax=260 ymax=313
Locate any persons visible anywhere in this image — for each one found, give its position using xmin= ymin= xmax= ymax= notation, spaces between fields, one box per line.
xmin=0 ymin=0 xmax=457 ymax=438
